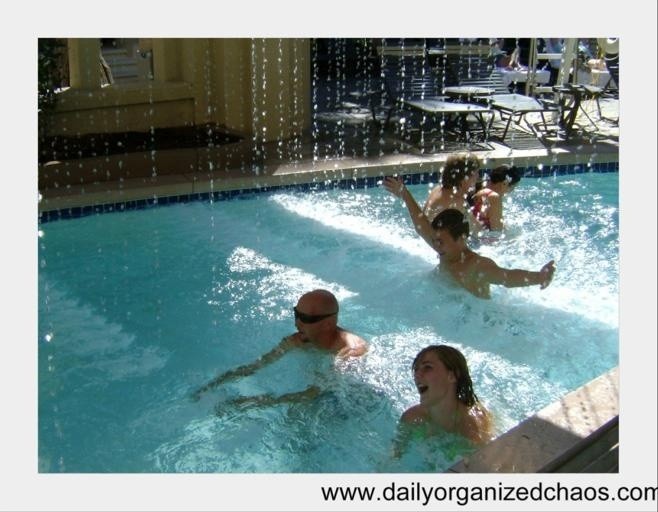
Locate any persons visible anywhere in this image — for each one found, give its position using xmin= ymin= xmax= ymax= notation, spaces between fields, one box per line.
xmin=383 ymin=150 xmax=556 ymax=300
xmin=190 ymin=288 xmax=367 ymax=417
xmin=389 ymin=345 xmax=492 ymax=459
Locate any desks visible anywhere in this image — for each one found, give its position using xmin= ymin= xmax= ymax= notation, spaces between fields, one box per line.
xmin=396 ymin=64 xmax=612 ymax=152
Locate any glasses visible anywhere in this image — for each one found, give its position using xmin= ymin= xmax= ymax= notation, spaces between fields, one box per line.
xmin=292 ymin=306 xmax=338 ymax=325
